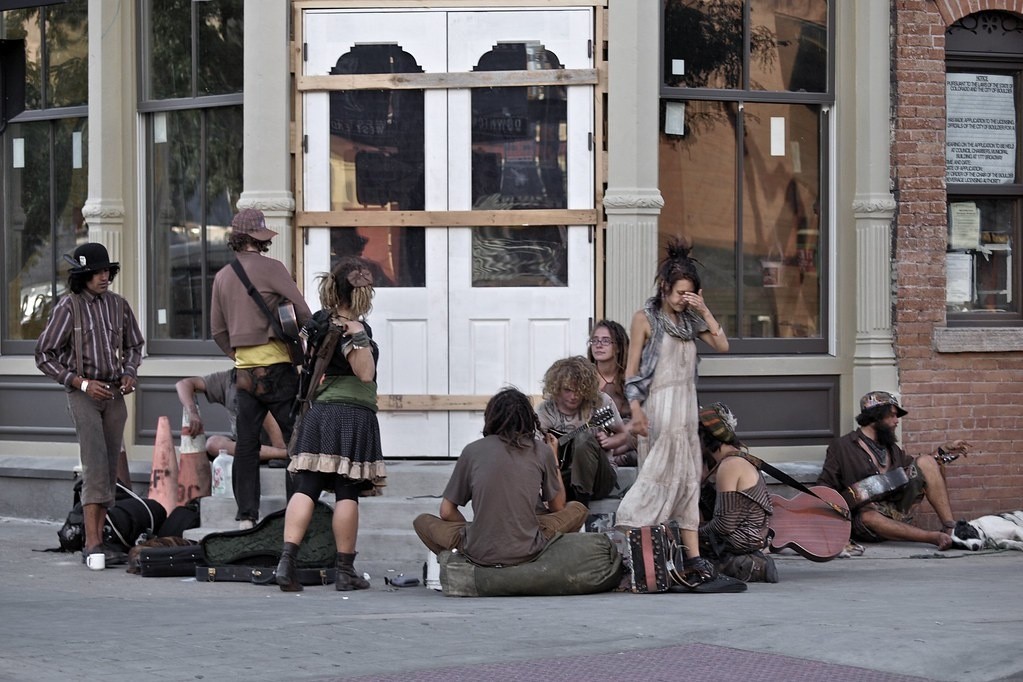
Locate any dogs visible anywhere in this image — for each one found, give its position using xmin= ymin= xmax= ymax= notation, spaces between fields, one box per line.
xmin=950 ymin=509 xmax=1023 ymax=552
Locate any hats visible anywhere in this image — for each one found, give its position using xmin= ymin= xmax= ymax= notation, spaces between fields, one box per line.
xmin=859 ymin=390 xmax=908 ymax=418
xmin=232 ymin=208 xmax=279 ymax=241
xmin=63 ymin=243 xmax=119 ymax=273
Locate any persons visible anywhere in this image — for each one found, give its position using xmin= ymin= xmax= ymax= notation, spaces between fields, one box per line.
xmin=414 ymin=388 xmax=588 ymax=566
xmin=274 ymin=255 xmax=388 ymax=591
xmin=692 ymin=402 xmax=780 ymax=584
xmin=814 ymin=390 xmax=972 ymax=552
xmin=586 ymin=320 xmax=636 ymax=469
xmin=623 ymin=242 xmax=731 ymax=589
xmin=535 ymin=354 xmax=623 ymax=509
xmin=36 ymin=243 xmax=145 ymax=563
xmin=175 ymin=209 xmax=313 ymax=468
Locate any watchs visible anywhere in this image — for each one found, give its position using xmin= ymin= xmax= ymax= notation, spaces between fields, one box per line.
xmin=710 ymin=323 xmax=722 ymax=336
xmin=80 ymin=378 xmax=89 ymax=394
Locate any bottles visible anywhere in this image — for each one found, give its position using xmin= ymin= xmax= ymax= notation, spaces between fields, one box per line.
xmin=211 ymin=448 xmax=233 ymax=498
xmin=426 ymin=549 xmax=442 ymax=590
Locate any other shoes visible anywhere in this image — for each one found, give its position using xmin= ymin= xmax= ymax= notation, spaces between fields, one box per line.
xmin=81 ymin=543 xmax=129 ymax=566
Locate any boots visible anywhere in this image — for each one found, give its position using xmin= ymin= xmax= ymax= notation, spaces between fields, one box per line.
xmin=275 ymin=543 xmax=302 ymax=591
xmin=336 ymin=552 xmax=371 ymax=591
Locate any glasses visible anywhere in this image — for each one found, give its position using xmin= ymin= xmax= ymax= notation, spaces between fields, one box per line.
xmin=589 ymin=338 xmax=615 ymax=346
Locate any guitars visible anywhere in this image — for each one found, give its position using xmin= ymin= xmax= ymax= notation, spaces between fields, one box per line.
xmin=767 ymin=486 xmax=851 ymax=563
xmin=278 ymin=299 xmax=305 ymax=367
xmin=841 ymin=452 xmax=960 ymax=511
xmin=546 ymin=406 xmax=615 ymax=473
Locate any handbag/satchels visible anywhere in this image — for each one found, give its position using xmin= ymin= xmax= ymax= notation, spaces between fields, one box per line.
xmin=57 ymin=484 xmax=168 ymax=552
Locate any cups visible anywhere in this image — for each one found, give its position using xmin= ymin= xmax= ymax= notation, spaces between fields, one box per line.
xmin=86 ymin=553 xmax=105 ymax=570
